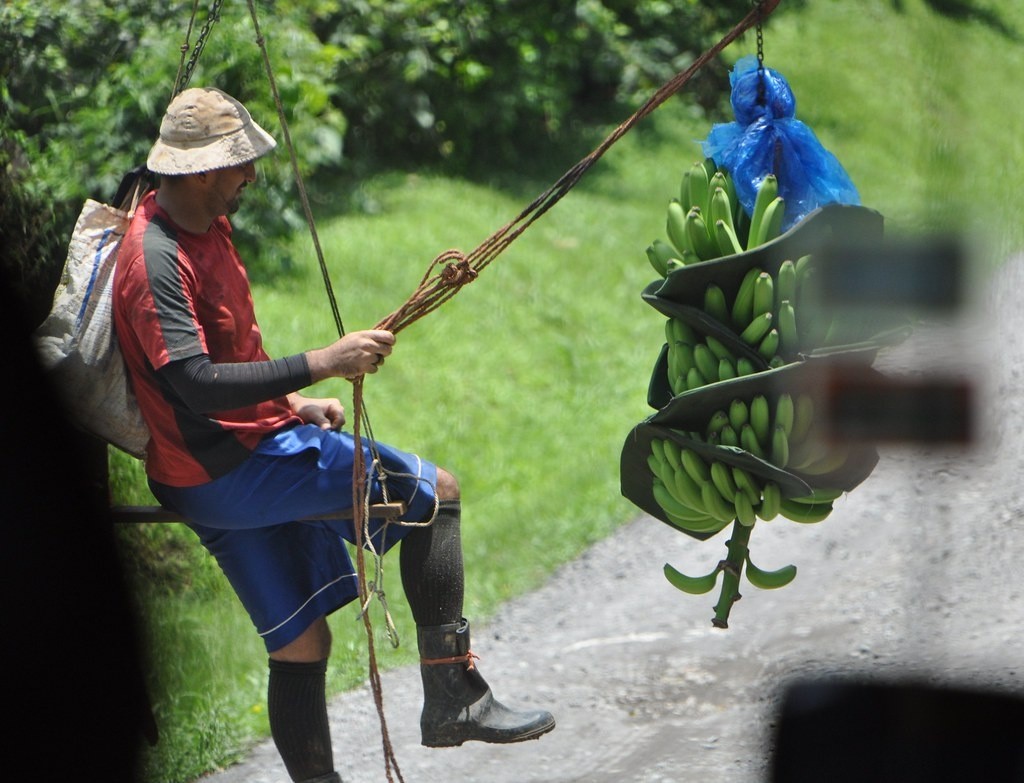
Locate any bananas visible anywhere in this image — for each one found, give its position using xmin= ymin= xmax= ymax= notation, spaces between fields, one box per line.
xmin=646 ymin=158 xmax=848 ymax=593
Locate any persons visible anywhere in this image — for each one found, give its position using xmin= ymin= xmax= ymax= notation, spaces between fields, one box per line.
xmin=111 ymin=86 xmax=557 ymax=783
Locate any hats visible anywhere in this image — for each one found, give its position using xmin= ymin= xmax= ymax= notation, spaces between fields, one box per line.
xmin=147 ymin=86 xmax=277 ymax=175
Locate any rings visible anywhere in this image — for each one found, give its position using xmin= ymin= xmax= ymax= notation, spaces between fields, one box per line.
xmin=373 ymin=354 xmax=380 ymax=365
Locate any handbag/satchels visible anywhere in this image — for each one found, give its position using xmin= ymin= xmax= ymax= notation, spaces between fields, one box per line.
xmin=33 ymin=164 xmax=155 ymax=460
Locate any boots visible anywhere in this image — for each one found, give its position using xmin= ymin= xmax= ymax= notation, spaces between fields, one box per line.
xmin=294 ymin=771 xmax=344 ymax=783
xmin=416 ymin=617 xmax=556 ymax=748
